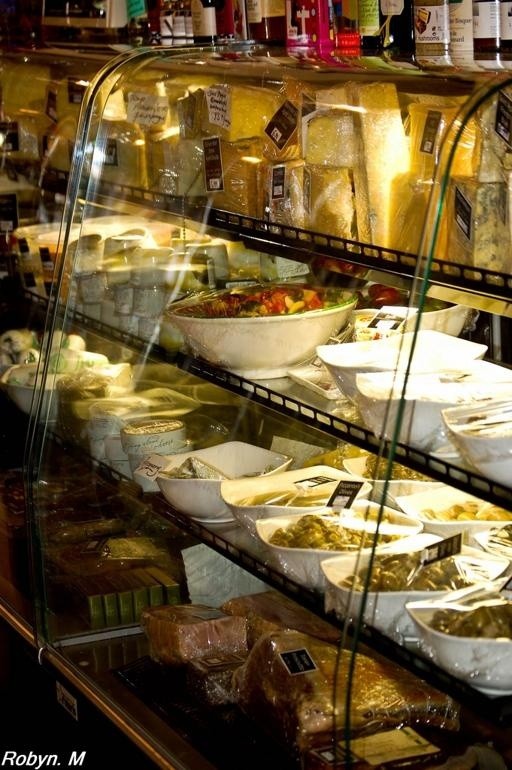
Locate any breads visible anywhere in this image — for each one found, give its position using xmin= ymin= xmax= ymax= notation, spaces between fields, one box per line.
xmin=220 ymin=589 xmax=351 ymax=652
xmin=142 ymin=604 xmax=250 ymax=663
xmin=102 ymin=73 xmax=512 ymax=289
xmin=237 ymin=631 xmax=461 ymax=759
xmin=0 ymin=55 xmax=101 ymax=171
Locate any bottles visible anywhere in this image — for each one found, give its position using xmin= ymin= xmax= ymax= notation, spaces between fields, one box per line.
xmin=134 ymin=69 xmax=170 ymax=132
xmin=447 ymin=0 xmax=478 ymax=68
xmin=499 ymin=0 xmax=512 ymax=70
xmin=473 ymin=0 xmax=503 ymax=69
xmin=183 ymin=1 xmax=194 ymax=39
xmin=160 ymin=1 xmax=173 ymax=38
xmin=358 ymin=0 xmax=387 ymax=56
xmin=262 ymin=0 xmax=288 ymax=44
xmin=189 ymin=0 xmax=217 ymax=44
xmin=415 ymin=1 xmax=453 ymax=69
xmin=244 ymin=0 xmax=266 ymax=44
xmin=173 ymin=0 xmax=186 ymax=38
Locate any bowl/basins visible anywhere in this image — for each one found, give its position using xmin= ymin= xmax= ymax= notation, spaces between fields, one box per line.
xmin=473 ymin=526 xmax=512 ymax=558
xmin=404 ymin=593 xmax=512 ymax=698
xmin=149 ymin=440 xmax=294 ymax=524
xmin=314 ymin=327 xmax=487 ymax=410
xmin=253 ymin=497 xmax=423 ymax=593
xmin=341 ymin=454 xmax=446 ymax=501
xmin=440 ymin=402 xmax=511 ymax=487
xmin=164 ymin=278 xmax=358 ymax=382
xmin=355 ymin=360 xmax=512 ymax=446
xmin=217 ymin=462 xmax=373 ymax=539
xmin=393 ymin=489 xmax=510 ymax=537
xmin=317 ymin=533 xmax=511 ymax=633
xmin=3 ymin=381 xmax=59 ymax=424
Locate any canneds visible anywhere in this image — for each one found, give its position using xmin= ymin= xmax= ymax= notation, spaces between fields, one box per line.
xmin=121 ymin=419 xmax=187 ymax=454
xmin=129 ymin=440 xmax=192 ymax=472
